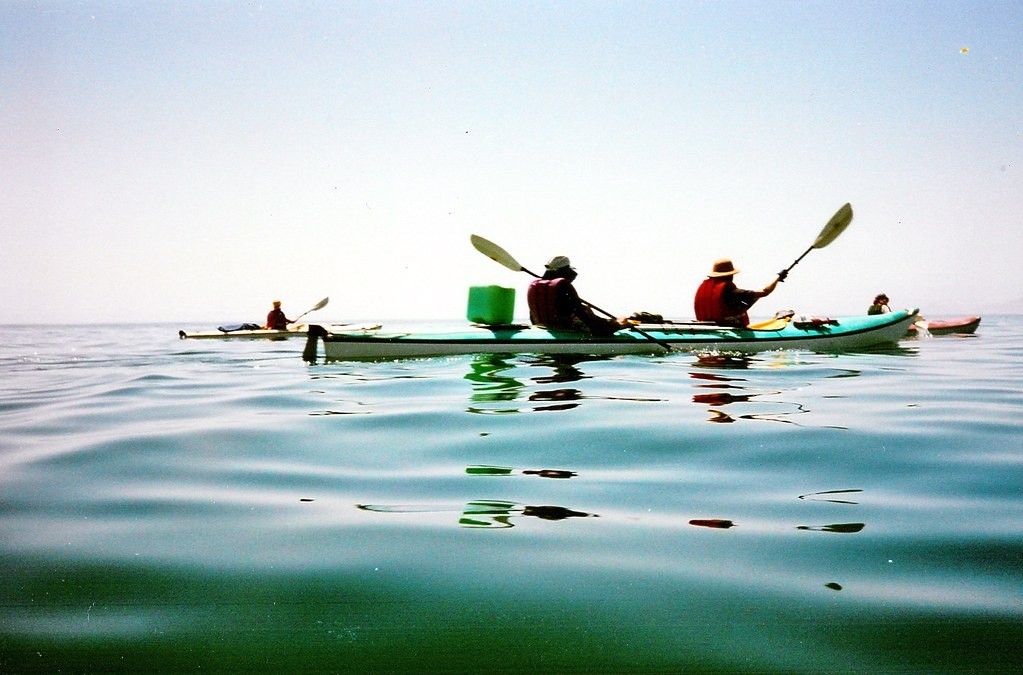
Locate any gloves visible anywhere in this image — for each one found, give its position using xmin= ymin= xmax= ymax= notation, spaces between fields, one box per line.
xmin=778 ymin=269 xmax=788 ymax=278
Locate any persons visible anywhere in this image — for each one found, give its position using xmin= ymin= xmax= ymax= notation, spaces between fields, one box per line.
xmin=266 ymin=300 xmax=295 ymax=330
xmin=527 ymin=256 xmax=629 ymax=335
xmin=694 ymin=260 xmax=789 ymax=328
xmin=868 ymin=294 xmax=891 ymax=315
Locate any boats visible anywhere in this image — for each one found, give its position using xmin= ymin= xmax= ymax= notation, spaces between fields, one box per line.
xmin=316 ymin=306 xmax=921 ymax=362
xmin=179 ymin=325 xmax=382 ymax=341
xmin=907 ymin=313 xmax=982 ymax=334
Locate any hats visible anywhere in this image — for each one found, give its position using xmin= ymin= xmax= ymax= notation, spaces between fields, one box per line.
xmin=273 ymin=300 xmax=282 ymax=306
xmin=545 ymin=256 xmax=576 ymax=271
xmin=707 ymin=259 xmax=740 ymax=277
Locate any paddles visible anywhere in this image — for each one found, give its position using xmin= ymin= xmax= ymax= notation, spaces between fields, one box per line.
xmin=768 ymin=203 xmax=857 ymax=293
xmin=750 ymin=309 xmax=792 ymax=330
xmin=291 ymin=296 xmax=332 ymax=324
xmin=469 ymin=235 xmax=675 ymax=353
